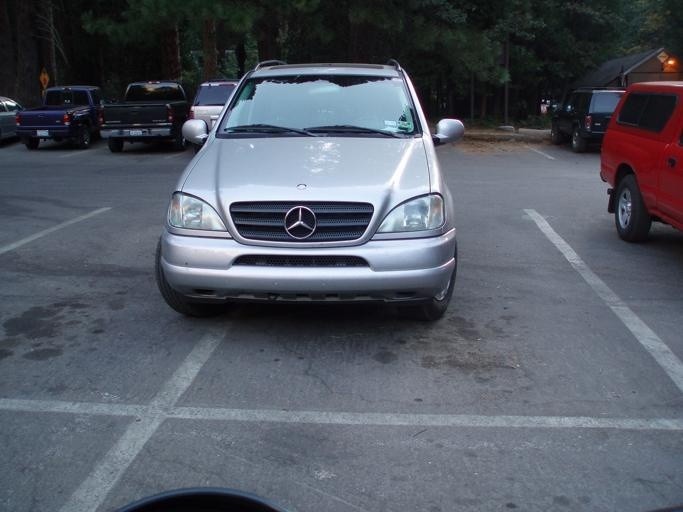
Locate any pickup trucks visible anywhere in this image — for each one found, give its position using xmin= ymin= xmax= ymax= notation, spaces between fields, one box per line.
xmin=0 ymin=81 xmax=190 ymax=153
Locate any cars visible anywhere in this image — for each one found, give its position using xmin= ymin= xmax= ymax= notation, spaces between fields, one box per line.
xmin=550 ymin=81 xmax=682 ymax=244
